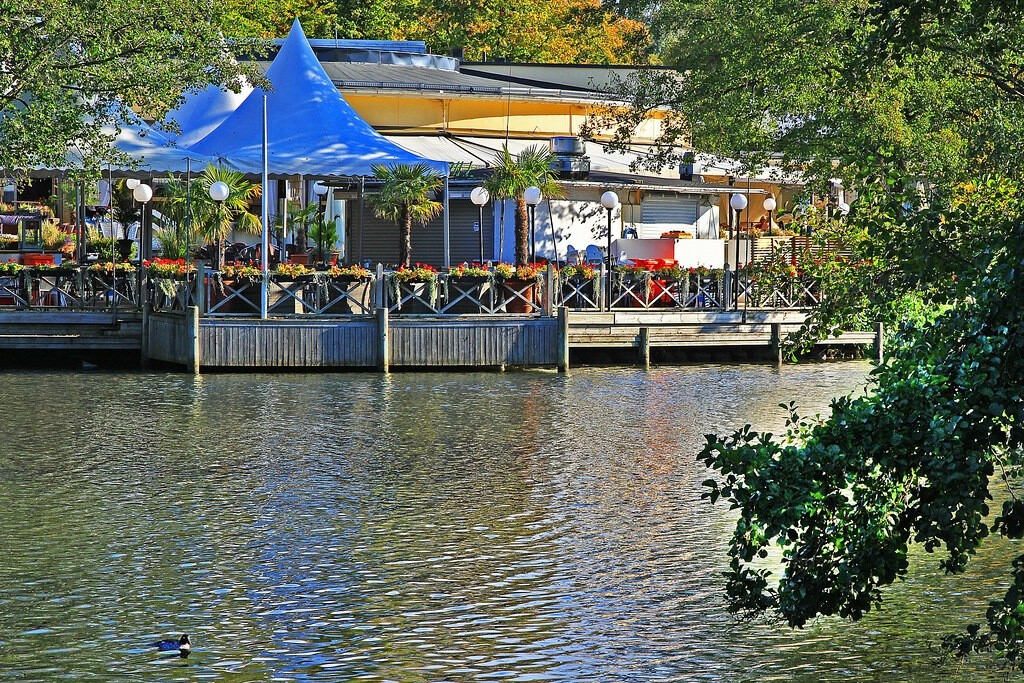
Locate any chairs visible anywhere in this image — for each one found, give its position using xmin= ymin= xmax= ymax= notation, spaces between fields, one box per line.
xmin=0 ymin=276 xmax=19 ymax=306
xmin=41 ymin=276 xmax=73 ymax=305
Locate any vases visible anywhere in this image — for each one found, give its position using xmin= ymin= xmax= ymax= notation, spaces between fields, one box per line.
xmin=651 ymin=275 xmax=670 ymax=280
xmin=615 ymin=273 xmax=647 ymax=292
xmin=214 ymin=274 xmax=263 ymax=282
xmin=393 ymin=276 xmax=429 ymax=283
xmin=0 ymin=271 xmax=129 ymax=277
xmin=564 ymin=273 xmax=595 ymax=307
xmin=151 ymin=271 xmax=195 ymax=280
xmin=691 ymin=275 xmax=715 ymax=279
xmin=451 ymin=276 xmax=488 ymax=283
xmin=328 ymin=275 xmax=369 ymax=283
xmin=497 ymin=273 xmax=539 ymax=313
xmin=272 ymin=274 xmax=316 ymax=282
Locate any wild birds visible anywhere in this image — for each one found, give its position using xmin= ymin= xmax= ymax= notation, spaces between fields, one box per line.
xmin=145 ymin=633 xmax=192 ymax=651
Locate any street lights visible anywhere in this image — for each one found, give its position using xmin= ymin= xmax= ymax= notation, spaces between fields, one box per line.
xmin=600 ymin=190 xmax=619 ymax=311
xmin=470 ymin=186 xmax=490 ymax=265
xmin=763 ymin=198 xmax=777 ymax=306
xmin=312 ymin=179 xmax=329 ymax=267
xmin=523 ymin=186 xmax=543 ymax=313
xmin=133 ymin=183 xmax=153 ymax=266
xmin=730 ymin=194 xmax=748 ymax=311
xmin=209 ymin=181 xmax=230 ymax=268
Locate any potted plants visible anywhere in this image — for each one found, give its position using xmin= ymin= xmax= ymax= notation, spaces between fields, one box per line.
xmin=289 ymin=200 xmax=338 ymax=267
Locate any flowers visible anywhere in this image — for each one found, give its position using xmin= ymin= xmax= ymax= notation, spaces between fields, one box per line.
xmin=386 ymin=262 xmax=439 ymax=311
xmin=328 ymin=264 xmax=369 ymax=280
xmin=496 ymin=260 xmax=548 ymax=280
xmin=561 ymin=261 xmax=600 ymax=300
xmin=33 ymin=260 xmax=78 ymax=271
xmin=614 ymin=265 xmax=650 ymax=299
xmin=276 ymin=260 xmax=313 ymax=279
xmin=220 ymin=260 xmax=263 ymax=277
xmin=0 ymin=257 xmax=21 ymax=275
xmin=143 ymin=257 xmax=196 ymax=275
xmin=91 ymin=262 xmax=131 ymax=271
xmin=443 ymin=261 xmax=500 ymax=305
xmin=653 ymin=260 xmax=725 ymax=302
